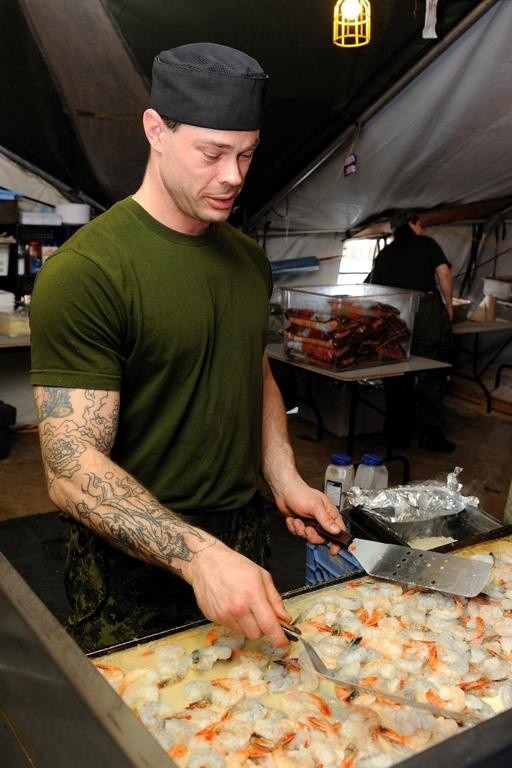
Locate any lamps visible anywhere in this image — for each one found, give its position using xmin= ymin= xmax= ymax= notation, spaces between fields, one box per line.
xmin=332 ymin=1 xmax=371 ymax=49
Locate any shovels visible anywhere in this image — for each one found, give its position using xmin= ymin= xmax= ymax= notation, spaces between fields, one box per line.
xmin=277 ymin=617 xmax=464 ymax=720
xmin=295 ymin=516 xmax=492 ymax=598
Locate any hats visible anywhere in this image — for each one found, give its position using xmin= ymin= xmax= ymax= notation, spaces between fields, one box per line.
xmin=148 ymin=41 xmax=272 ymax=132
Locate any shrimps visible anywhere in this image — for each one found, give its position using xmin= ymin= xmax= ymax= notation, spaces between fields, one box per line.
xmin=92 ymin=535 xmax=512 ymax=768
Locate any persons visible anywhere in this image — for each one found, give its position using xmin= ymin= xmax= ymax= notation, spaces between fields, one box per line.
xmin=28 ymin=41 xmax=350 ymax=657
xmin=369 ymin=211 xmax=459 ymax=453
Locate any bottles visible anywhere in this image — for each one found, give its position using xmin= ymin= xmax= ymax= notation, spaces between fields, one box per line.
xmin=323 ymin=451 xmax=355 ymax=514
xmin=353 ymin=453 xmax=389 ymax=493
